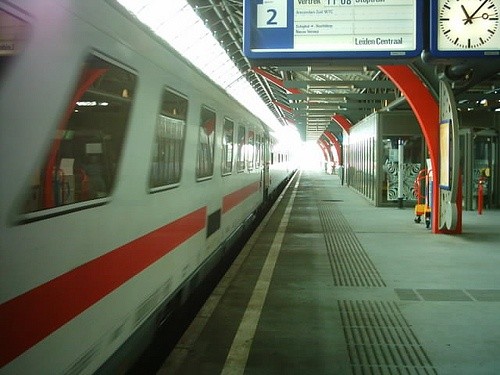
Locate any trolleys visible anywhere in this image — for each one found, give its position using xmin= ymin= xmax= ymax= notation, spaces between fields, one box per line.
xmin=414 ymin=168 xmax=427 ymax=224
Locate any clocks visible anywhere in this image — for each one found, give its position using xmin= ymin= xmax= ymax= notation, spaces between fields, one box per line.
xmin=429 ymin=0 xmax=500 ymax=60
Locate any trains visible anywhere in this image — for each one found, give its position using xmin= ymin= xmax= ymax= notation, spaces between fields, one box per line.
xmin=1 ymin=0 xmax=297 ymax=375
